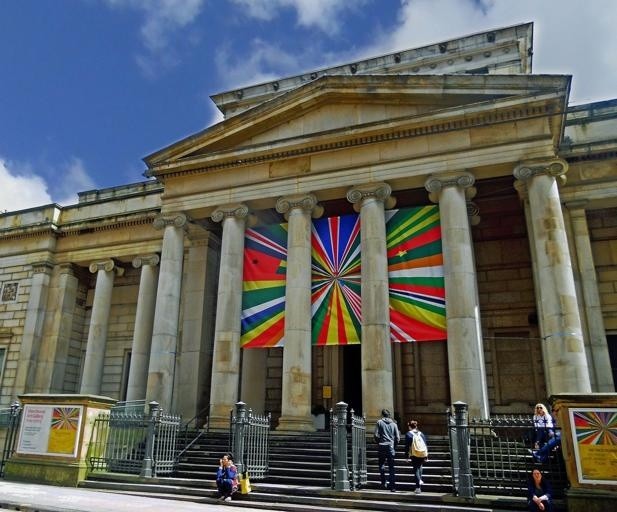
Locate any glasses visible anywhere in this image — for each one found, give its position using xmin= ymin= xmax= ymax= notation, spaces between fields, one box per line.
xmin=537 ymin=407 xmax=542 ymax=409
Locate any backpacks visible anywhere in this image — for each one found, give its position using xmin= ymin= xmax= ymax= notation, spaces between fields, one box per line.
xmin=410 ymin=430 xmax=428 ymax=459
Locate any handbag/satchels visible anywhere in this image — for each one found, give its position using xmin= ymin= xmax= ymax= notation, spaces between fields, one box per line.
xmin=238 ymin=471 xmax=251 ymax=494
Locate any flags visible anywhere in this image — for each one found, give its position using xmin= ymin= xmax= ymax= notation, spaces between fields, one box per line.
xmin=239 ymin=204 xmax=448 ymax=349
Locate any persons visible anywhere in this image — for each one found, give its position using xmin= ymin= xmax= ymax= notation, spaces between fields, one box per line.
xmin=526 ymin=469 xmax=555 ymax=512
xmin=217 ymin=455 xmax=239 ymax=502
xmin=375 ymin=410 xmax=401 ymax=492
xmin=405 ymin=421 xmax=429 ymax=494
xmin=529 ymin=403 xmax=562 ymax=464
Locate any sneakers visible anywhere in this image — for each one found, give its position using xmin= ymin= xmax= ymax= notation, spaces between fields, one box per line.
xmin=224 ymin=496 xmax=231 ymax=502
xmin=217 ymin=495 xmax=225 ymax=502
xmin=414 ymin=487 xmax=421 ymax=494
xmin=419 ymin=479 xmax=424 ymax=485
